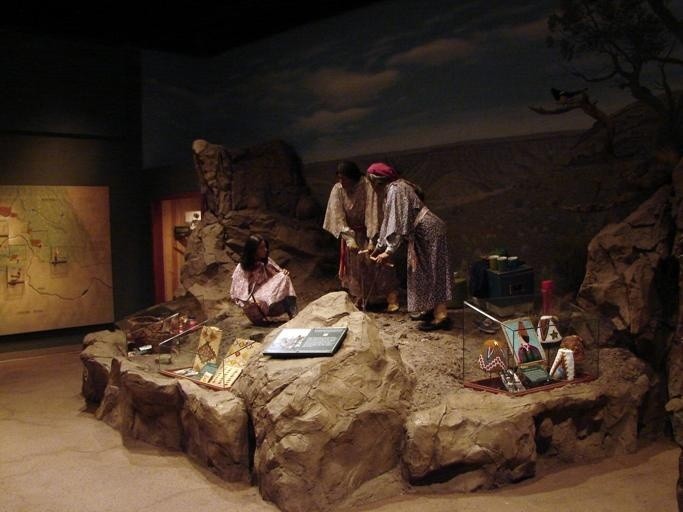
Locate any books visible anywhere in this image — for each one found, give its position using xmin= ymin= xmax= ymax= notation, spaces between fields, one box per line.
xmin=218 ymin=338 xmax=256 ymax=373
xmin=192 ymin=362 xmax=243 ymax=389
xmin=191 ymin=325 xmax=222 ymax=372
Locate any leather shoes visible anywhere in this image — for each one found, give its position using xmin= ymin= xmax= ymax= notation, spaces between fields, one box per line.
xmin=408 ymin=309 xmax=454 ymax=331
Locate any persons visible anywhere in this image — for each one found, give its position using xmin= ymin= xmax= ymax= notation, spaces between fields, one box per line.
xmin=228 ymin=233 xmax=298 ymax=324
xmin=365 ymin=162 xmax=454 ymax=331
xmin=321 ymin=157 xmax=401 ymax=314
